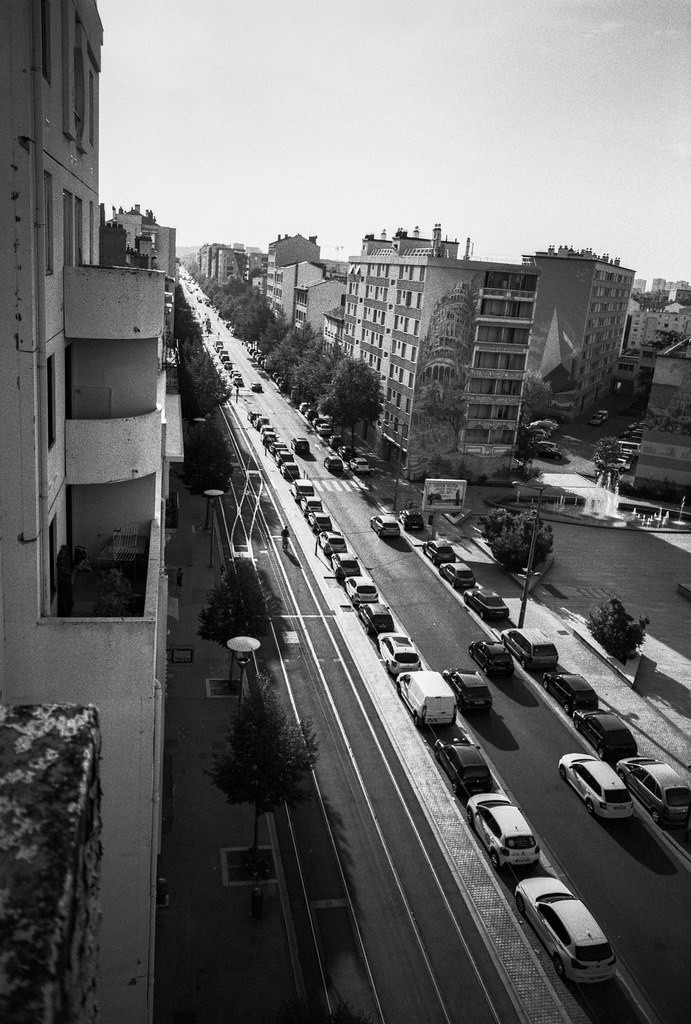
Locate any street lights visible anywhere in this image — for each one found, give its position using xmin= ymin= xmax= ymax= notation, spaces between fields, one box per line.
xmin=225 ymin=637 xmax=261 ymax=712
xmin=204 ymin=490 xmax=224 ymax=568
xmin=513 ymin=480 xmax=551 ymax=628
xmin=382 ymin=418 xmax=404 ymax=512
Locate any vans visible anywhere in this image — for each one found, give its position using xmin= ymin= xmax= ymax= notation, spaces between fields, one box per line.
xmin=396 ymin=672 xmax=457 ymax=730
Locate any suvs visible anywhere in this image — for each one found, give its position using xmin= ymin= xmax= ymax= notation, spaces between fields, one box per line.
xmin=500 ymin=628 xmax=560 ymax=672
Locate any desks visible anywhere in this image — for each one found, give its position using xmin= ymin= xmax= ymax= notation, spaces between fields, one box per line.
xmin=109 ymin=535 xmax=147 ymax=587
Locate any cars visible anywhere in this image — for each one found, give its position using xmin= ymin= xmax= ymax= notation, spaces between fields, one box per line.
xmin=358 ymin=603 xmax=394 ymax=635
xmin=438 ymin=563 xmax=474 ymax=588
xmin=589 ymin=409 xmax=608 ymax=427
xmin=443 ymin=668 xmax=492 ymax=716
xmin=463 ymin=587 xmax=509 ymax=622
xmin=542 ymin=672 xmax=599 ymax=715
xmin=330 ymin=550 xmax=362 ymax=578
xmin=213 ymin=339 xmax=368 ymax=554
xmin=513 ymin=876 xmax=619 ymax=985
xmin=377 ymin=632 xmax=422 ymax=679
xmin=558 ymin=753 xmax=635 ymax=820
xmin=595 ymin=418 xmax=647 ymax=474
xmin=436 ymin=734 xmax=493 ymax=799
xmin=468 ymin=640 xmax=516 ymax=679
xmin=399 ymin=510 xmax=424 ymax=531
xmin=572 ymin=709 xmax=638 ymax=762
xmin=370 ymin=515 xmax=401 ymax=538
xmin=615 ymin=757 xmax=691 ymax=825
xmin=344 ymin=577 xmax=379 ymax=605
xmin=466 ymin=792 xmax=540 ymax=872
xmin=525 ymin=418 xmax=564 ymax=460
xmin=425 ymin=540 xmax=458 ymax=564
